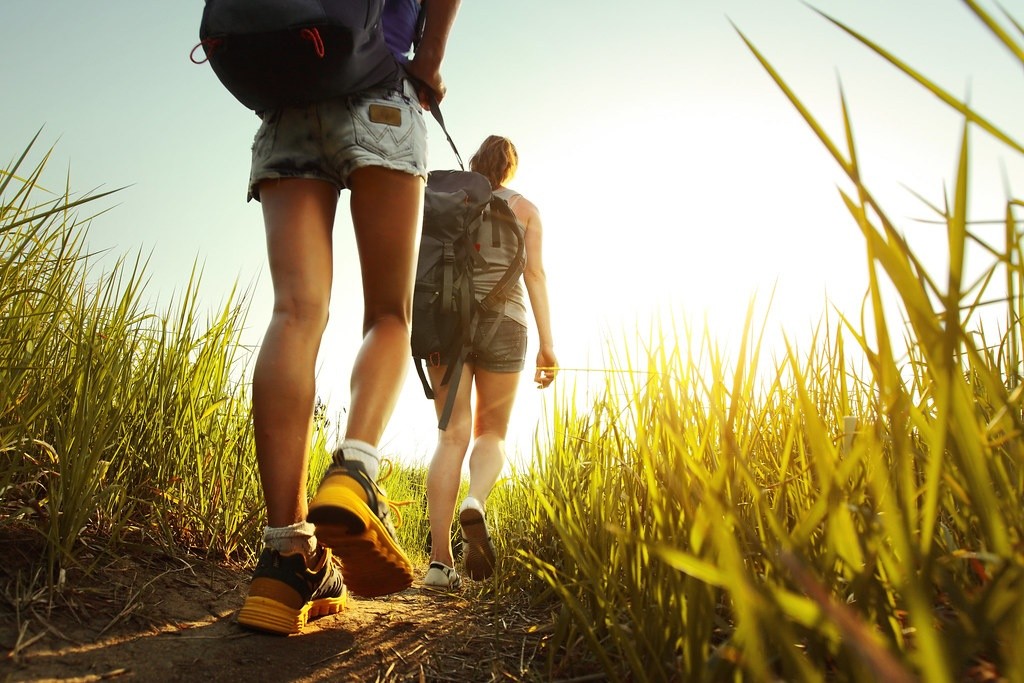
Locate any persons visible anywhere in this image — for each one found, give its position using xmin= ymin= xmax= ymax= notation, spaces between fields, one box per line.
xmin=238 ymin=0 xmax=462 ymax=633
xmin=425 ymin=134 xmax=560 ymax=587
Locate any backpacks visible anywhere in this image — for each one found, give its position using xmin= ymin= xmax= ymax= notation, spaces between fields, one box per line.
xmin=410 ymin=169 xmax=525 ymax=362
xmin=192 ymin=0 xmax=403 ymax=128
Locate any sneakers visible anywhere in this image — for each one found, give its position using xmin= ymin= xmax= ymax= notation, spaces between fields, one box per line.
xmin=459 ymin=498 xmax=496 ymax=581
xmin=306 ymin=448 xmax=413 ymax=598
xmin=418 ymin=560 xmax=460 ymax=594
xmin=238 ymin=540 xmax=348 ymax=634
